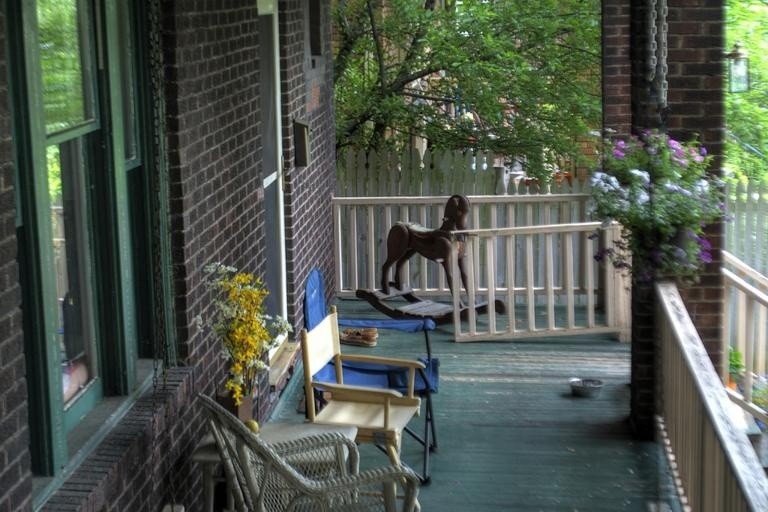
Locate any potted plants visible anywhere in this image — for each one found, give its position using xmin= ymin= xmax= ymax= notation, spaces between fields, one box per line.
xmin=728 ymin=346 xmax=745 ymax=390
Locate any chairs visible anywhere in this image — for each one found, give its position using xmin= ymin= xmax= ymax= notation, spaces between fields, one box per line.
xmin=300 ymin=305 xmax=425 ymax=512
xmin=303 ymin=267 xmax=440 ymax=484
xmin=197 ymin=393 xmax=420 ymax=512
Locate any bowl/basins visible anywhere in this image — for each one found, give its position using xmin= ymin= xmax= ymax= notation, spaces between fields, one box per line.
xmin=568 ymin=378 xmax=604 ymax=397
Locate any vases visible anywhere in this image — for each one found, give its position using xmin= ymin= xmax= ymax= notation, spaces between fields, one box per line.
xmin=216 ymin=391 xmax=253 ymax=429
xmin=630 ymin=226 xmax=685 ymax=278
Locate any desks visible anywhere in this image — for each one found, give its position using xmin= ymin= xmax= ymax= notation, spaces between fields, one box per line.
xmin=192 ymin=422 xmax=358 ymax=512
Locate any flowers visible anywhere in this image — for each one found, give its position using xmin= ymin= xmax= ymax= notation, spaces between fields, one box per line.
xmin=588 ymin=124 xmax=727 ymax=283
xmin=196 ymin=262 xmax=294 ymax=407
